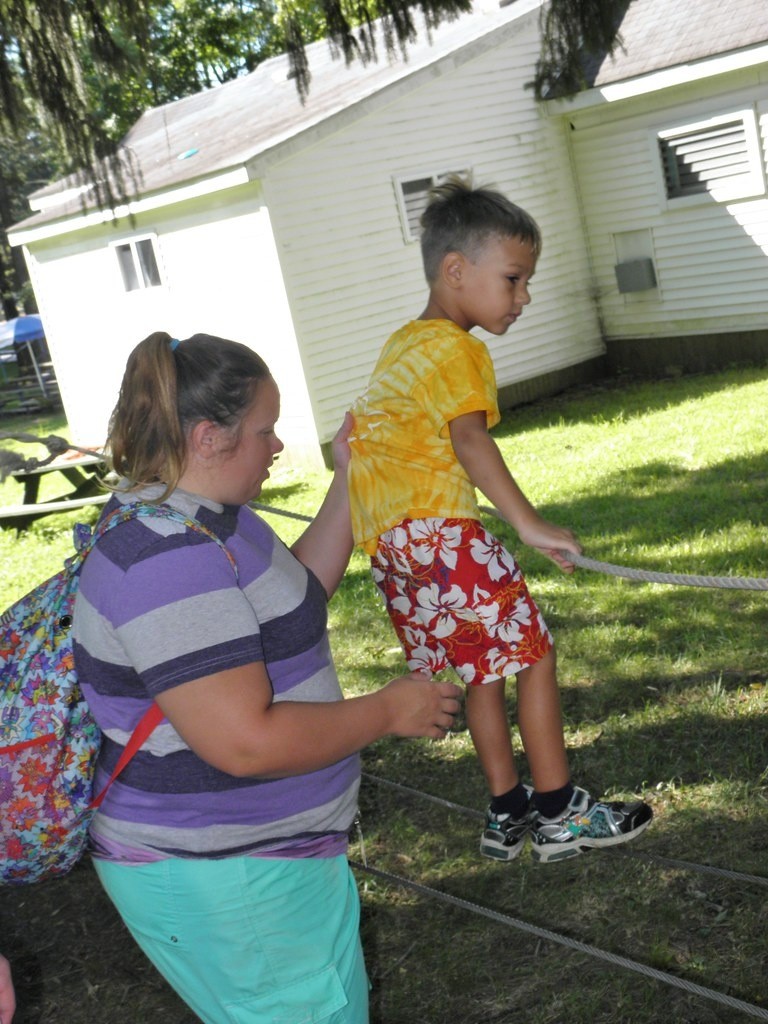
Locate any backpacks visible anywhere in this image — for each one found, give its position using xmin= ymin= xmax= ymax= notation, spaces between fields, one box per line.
xmin=0 ymin=504 xmax=237 ymax=885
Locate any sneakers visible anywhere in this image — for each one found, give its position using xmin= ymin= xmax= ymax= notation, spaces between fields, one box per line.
xmin=533 ymin=786 xmax=654 ymax=864
xmin=478 ymin=784 xmax=536 ymax=862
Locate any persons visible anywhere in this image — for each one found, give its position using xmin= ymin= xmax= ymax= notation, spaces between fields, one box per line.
xmin=72 ymin=331 xmax=462 ymax=1024
xmin=347 ymin=190 xmax=656 ymax=865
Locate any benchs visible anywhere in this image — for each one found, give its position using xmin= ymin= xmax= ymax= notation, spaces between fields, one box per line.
xmin=0 ymin=363 xmax=60 ymax=416
